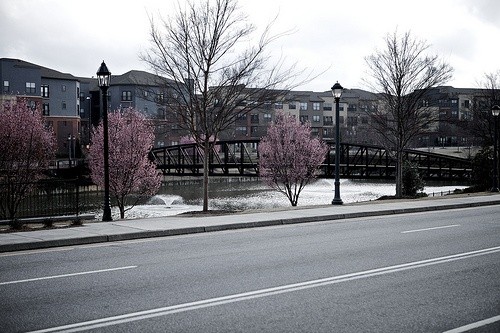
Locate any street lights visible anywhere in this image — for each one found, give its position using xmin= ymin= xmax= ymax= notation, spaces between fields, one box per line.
xmin=95 ymin=60 xmax=114 ymax=222
xmin=489 ymin=101 xmax=500 ymax=192
xmin=329 ymin=80 xmax=345 ymax=205
